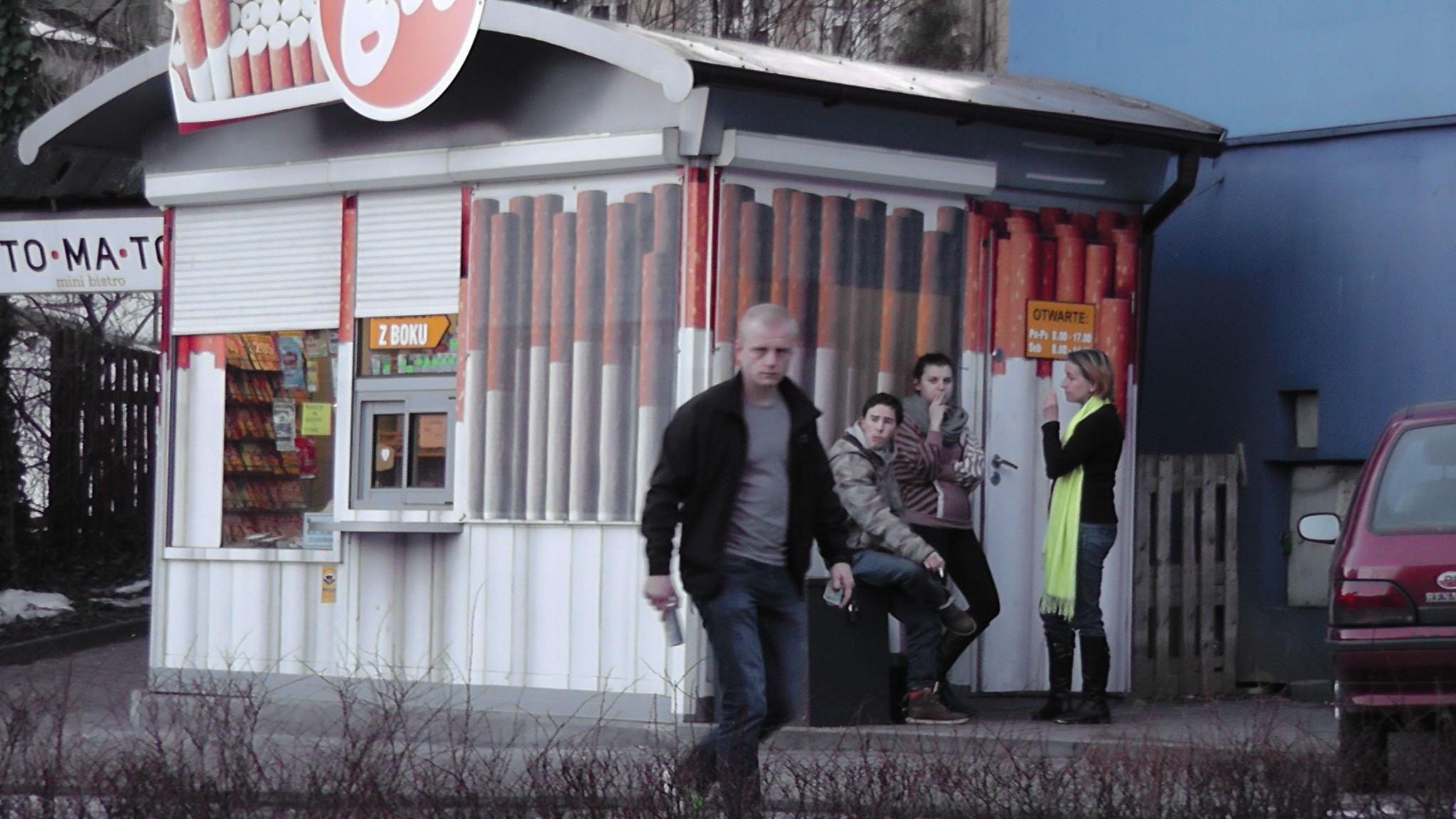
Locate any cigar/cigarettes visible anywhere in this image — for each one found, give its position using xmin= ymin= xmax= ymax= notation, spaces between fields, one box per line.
xmin=939 ymin=567 xmax=943 ymax=578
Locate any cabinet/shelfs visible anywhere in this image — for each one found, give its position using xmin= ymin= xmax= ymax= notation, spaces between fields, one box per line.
xmin=223 ymin=365 xmax=316 ymax=513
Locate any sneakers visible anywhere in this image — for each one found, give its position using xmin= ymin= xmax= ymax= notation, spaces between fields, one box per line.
xmin=940 ymin=596 xmax=976 ymax=636
xmin=905 ymin=691 xmax=969 ymax=724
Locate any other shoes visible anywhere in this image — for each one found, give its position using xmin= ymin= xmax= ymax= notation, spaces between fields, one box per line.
xmin=662 ymin=766 xmax=690 ymax=819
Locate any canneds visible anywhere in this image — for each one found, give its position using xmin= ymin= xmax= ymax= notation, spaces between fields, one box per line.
xmin=661 ymin=606 xmax=683 ymax=647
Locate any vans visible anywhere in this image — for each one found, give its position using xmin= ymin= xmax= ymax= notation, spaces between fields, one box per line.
xmin=1297 ymin=398 xmax=1456 ymax=794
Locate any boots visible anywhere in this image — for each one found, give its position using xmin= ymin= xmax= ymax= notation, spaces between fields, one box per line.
xmin=1057 ymin=636 xmax=1111 ymax=725
xmin=1031 ymin=631 xmax=1075 ymax=720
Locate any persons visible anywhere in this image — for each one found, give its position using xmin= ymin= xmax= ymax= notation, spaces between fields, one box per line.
xmin=829 ymin=352 xmax=1003 ymax=722
xmin=1029 ymin=350 xmax=1123 ymax=724
xmin=641 ymin=304 xmax=857 ymax=818
